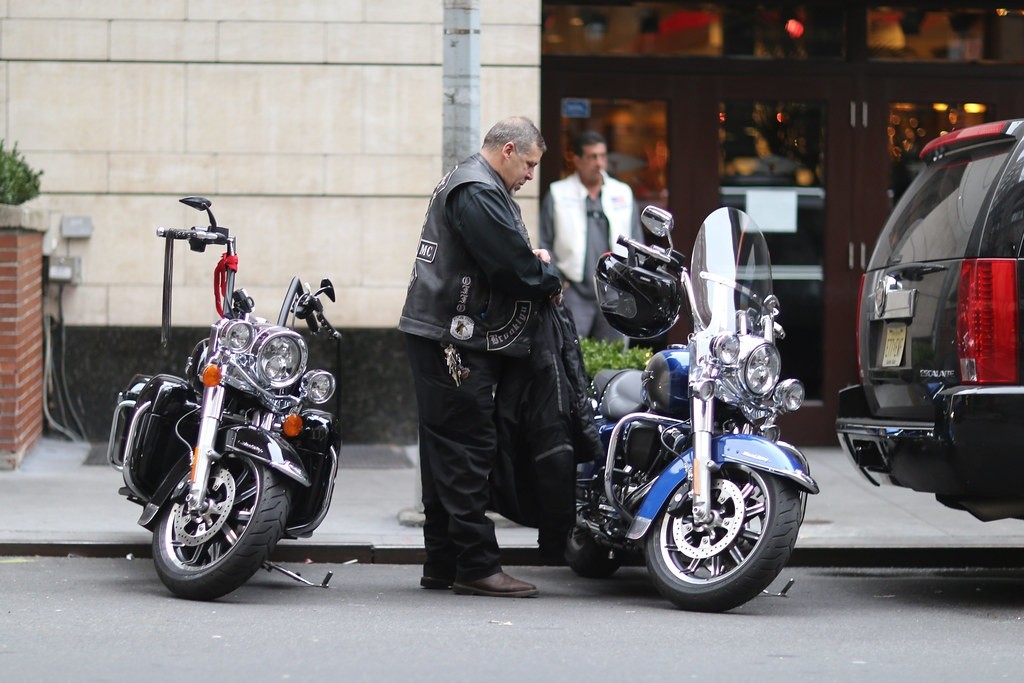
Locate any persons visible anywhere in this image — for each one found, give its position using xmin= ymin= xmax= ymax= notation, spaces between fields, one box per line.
xmin=396 ymin=116 xmax=564 ymax=597
xmin=541 ymin=130 xmax=644 ymax=352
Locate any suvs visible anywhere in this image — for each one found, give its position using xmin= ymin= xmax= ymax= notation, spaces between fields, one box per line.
xmin=832 ymin=118 xmax=1024 ymax=522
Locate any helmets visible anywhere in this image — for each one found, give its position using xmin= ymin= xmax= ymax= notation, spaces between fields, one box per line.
xmin=594 ymin=253 xmax=681 ymax=339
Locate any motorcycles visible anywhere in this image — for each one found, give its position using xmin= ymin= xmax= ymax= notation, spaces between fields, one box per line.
xmin=535 ymin=202 xmax=822 ymax=615
xmin=106 ymin=193 xmax=344 ymax=604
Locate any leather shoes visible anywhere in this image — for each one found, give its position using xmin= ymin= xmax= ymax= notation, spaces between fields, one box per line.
xmin=420 ymin=576 xmax=453 ymax=589
xmin=454 ymin=571 xmax=538 ymax=596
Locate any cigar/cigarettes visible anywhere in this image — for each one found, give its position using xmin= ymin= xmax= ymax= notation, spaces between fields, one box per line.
xmin=599 ymin=170 xmax=610 ymax=183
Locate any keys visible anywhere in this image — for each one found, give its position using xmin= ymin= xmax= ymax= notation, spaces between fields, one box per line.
xmin=444 ymin=345 xmax=469 ymax=387
